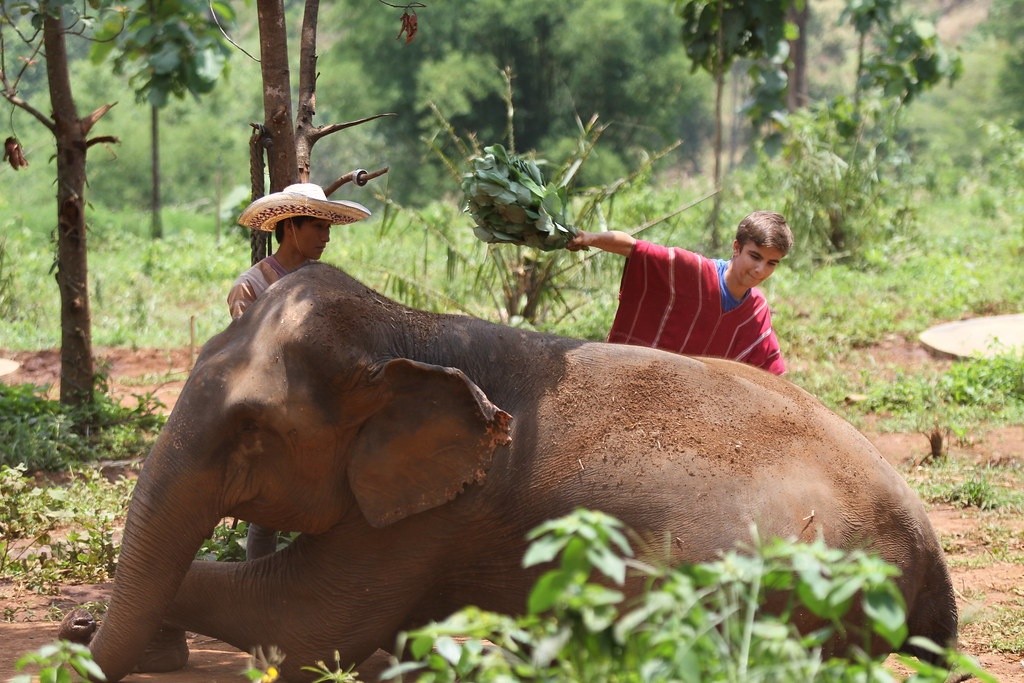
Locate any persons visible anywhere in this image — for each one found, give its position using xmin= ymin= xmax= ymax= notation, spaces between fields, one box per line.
xmin=567 ymin=210 xmax=795 ymax=377
xmin=226 ymin=182 xmax=371 ymax=325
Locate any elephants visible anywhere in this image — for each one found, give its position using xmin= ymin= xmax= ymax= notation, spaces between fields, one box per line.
xmin=81 ymin=262 xmax=957 ymax=683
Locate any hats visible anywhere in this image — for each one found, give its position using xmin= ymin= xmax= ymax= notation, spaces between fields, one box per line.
xmin=236 ymin=185 xmax=371 ymax=233
